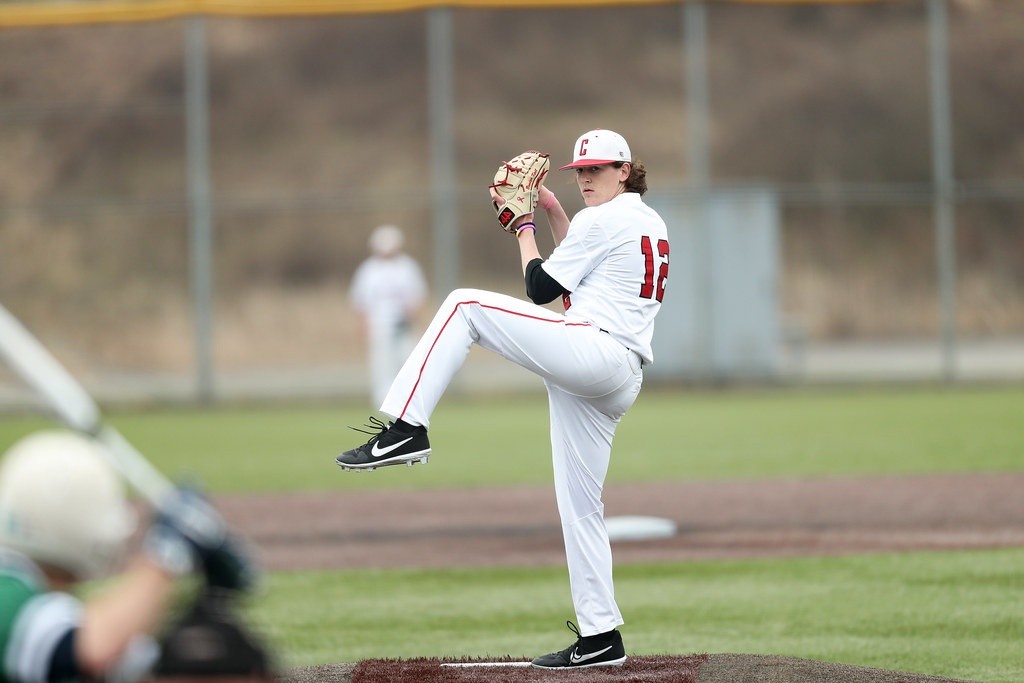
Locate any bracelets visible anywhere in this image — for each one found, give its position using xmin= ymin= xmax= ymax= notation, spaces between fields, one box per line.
xmin=543 ymin=192 xmax=555 ymax=210
xmin=514 ymin=222 xmax=536 ymax=236
xmin=516 ymin=224 xmax=536 ymax=239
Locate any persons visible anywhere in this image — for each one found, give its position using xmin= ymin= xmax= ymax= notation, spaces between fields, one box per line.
xmin=2 ymin=430 xmax=248 ymax=683
xmin=335 ymin=128 xmax=669 ymax=671
xmin=348 ymin=224 xmax=431 ymax=410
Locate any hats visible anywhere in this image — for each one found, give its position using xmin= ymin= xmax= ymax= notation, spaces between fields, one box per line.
xmin=558 ymin=127 xmax=631 ymax=171
xmin=0 ymin=429 xmax=139 ymax=581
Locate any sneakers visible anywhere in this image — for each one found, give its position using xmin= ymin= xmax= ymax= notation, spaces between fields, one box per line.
xmin=335 ymin=417 xmax=432 ymax=473
xmin=531 ymin=620 xmax=627 ymax=671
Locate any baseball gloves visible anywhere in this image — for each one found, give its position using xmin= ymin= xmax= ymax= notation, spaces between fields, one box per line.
xmin=486 ymin=149 xmax=553 ymax=232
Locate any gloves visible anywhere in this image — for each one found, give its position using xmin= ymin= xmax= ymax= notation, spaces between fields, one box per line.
xmin=198 ymin=540 xmax=250 ymax=595
xmin=144 ymin=481 xmax=221 ymax=576
